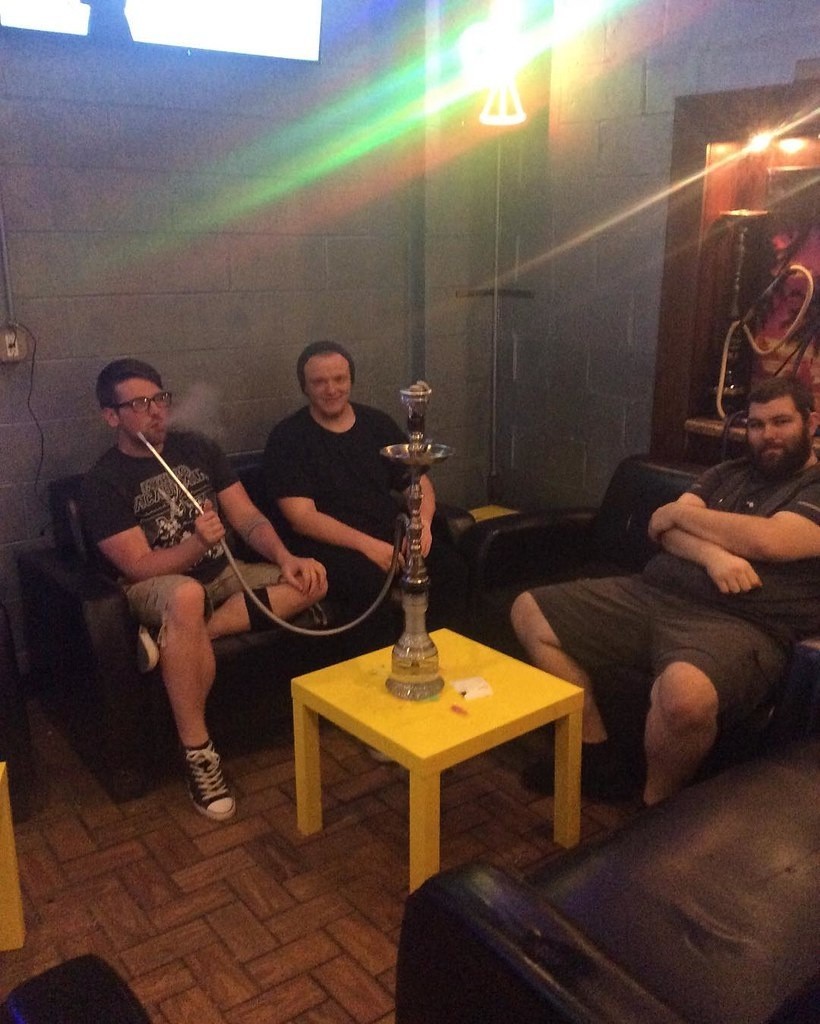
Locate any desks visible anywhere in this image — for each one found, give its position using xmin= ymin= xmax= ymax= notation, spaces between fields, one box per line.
xmin=288 ymin=629 xmax=587 ymax=896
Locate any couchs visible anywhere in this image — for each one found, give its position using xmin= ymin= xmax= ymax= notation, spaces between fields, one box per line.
xmin=394 ymin=639 xmax=820 ymax=1023
xmin=16 ymin=459 xmax=366 ymax=802
xmin=228 ymin=448 xmax=477 ymax=668
xmin=454 ymin=450 xmax=820 ymax=785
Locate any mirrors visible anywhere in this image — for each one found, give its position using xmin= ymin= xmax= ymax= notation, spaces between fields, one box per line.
xmin=645 ymin=73 xmax=819 ymax=475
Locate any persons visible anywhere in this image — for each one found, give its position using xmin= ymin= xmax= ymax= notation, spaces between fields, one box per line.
xmin=508 ymin=376 xmax=820 ymax=812
xmin=258 ymin=340 xmax=451 ymax=652
xmin=83 ymin=358 xmax=328 ymax=821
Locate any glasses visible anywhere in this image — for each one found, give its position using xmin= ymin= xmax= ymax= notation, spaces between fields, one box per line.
xmin=115 ymin=391 xmax=173 ymax=413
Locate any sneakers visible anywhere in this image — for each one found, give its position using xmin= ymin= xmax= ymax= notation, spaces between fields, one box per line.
xmin=134 ymin=629 xmax=161 ymax=673
xmin=179 ymin=736 xmax=237 ymax=821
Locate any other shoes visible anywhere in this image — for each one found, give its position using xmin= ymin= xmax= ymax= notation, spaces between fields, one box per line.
xmin=523 ymin=760 xmax=627 ymax=798
